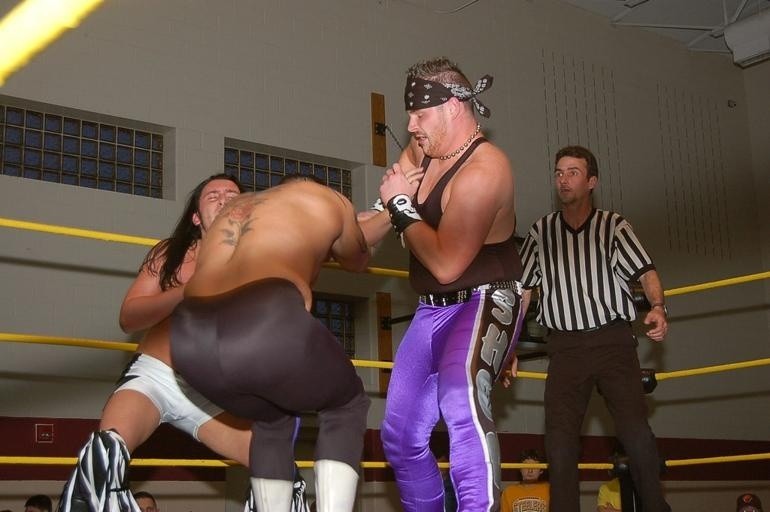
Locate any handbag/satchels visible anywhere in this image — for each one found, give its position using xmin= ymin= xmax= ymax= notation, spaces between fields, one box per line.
xmin=650 ymin=301 xmax=668 ymax=318
xmin=373 ymin=196 xmax=385 ymax=212
xmin=387 ymin=190 xmax=423 ymax=242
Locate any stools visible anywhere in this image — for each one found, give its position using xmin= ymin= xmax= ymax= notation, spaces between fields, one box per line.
xmin=418 ymin=279 xmax=526 ymax=307
xmin=546 ymin=315 xmax=625 ymax=338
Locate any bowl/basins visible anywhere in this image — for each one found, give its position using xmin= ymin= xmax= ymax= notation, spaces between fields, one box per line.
xmin=439 ymin=125 xmax=483 ymax=161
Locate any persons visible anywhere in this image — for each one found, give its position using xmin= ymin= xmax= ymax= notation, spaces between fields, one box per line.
xmin=596 ymin=441 xmax=626 ymax=511
xmin=55 ymin=135 xmax=428 ymax=511
xmin=378 ymin=53 xmax=528 ymax=511
xmin=133 ymin=490 xmax=157 ymax=512
xmin=735 ymin=492 xmax=764 ymax=512
xmin=499 ymin=449 xmax=552 ymax=512
xmin=493 ymin=143 xmax=671 ymax=511
xmin=168 ymin=160 xmax=426 ymax=512
xmin=24 ymin=494 xmax=53 ymax=512
